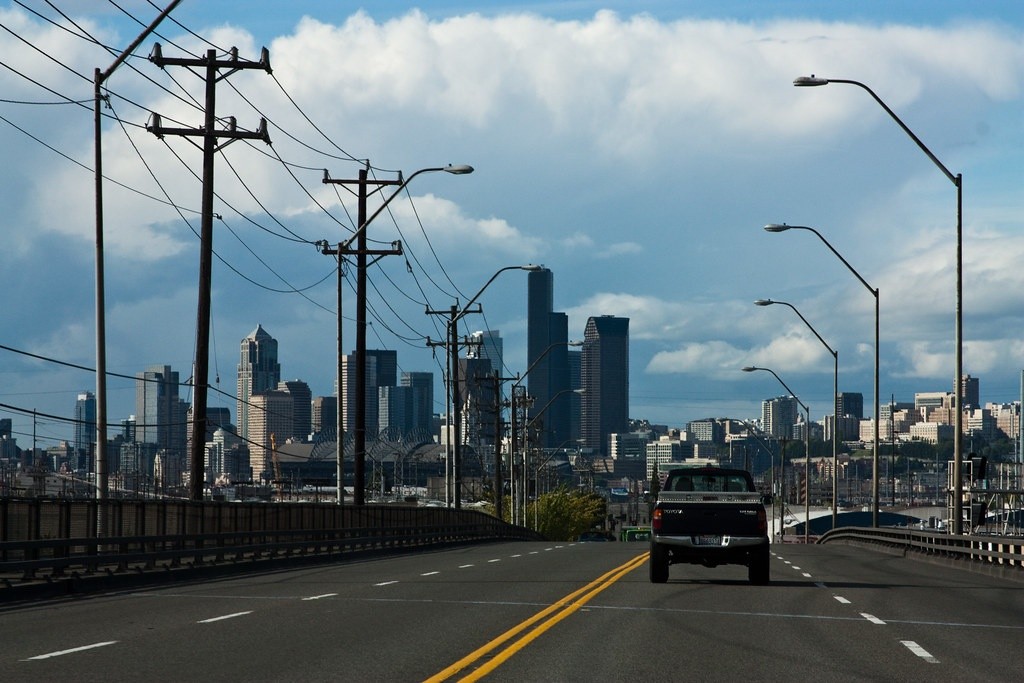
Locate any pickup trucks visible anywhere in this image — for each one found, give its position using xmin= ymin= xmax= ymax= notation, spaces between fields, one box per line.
xmin=644 ymin=464 xmax=775 ymax=585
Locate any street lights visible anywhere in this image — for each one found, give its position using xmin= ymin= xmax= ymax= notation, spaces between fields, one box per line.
xmin=716 ymin=416 xmax=775 ymax=546
xmin=533 ymin=439 xmax=587 ymax=531
xmin=509 ymin=339 xmax=584 ymax=526
xmin=754 ymin=299 xmax=837 ymax=531
xmin=334 ymin=165 xmax=476 ymax=491
xmin=792 ymin=76 xmax=963 ymax=537
xmin=763 ymin=221 xmax=881 ymax=527
xmin=443 ymin=265 xmax=543 ymax=508
xmin=740 ymin=365 xmax=810 ymax=544
xmin=522 ymin=389 xmax=587 ymax=524
